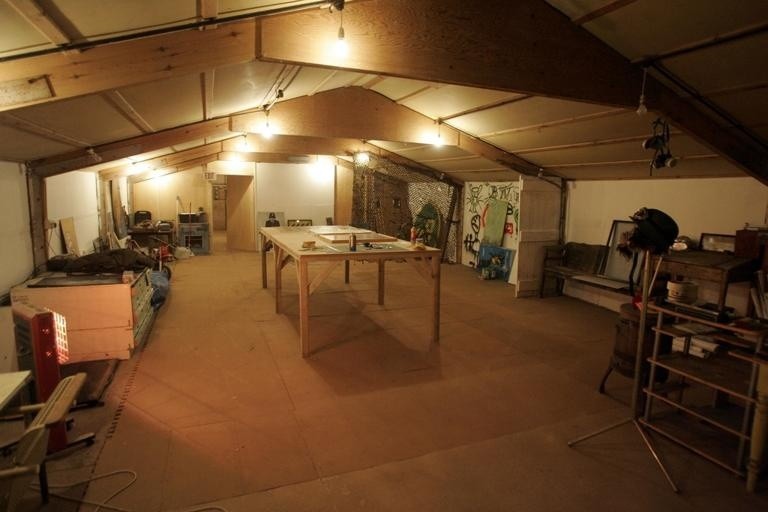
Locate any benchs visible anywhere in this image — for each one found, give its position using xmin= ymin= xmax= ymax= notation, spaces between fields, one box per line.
xmin=539 ymin=241 xmax=642 ymax=299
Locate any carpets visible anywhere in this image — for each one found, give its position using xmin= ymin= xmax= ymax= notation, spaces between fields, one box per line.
xmin=0 ymin=357 xmax=118 ymax=418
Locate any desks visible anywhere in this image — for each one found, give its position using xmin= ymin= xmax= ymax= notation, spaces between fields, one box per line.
xmin=258 ymin=224 xmax=444 ymax=359
xmin=652 ymin=249 xmax=757 ymax=317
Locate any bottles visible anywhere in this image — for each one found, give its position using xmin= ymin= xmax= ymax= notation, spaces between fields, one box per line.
xmin=411 ymin=223 xmax=417 ymax=245
xmin=349 ymin=232 xmax=356 ymax=251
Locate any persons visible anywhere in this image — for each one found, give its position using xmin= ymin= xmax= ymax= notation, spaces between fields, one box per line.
xmin=266 ymin=213 xmax=280 ymax=226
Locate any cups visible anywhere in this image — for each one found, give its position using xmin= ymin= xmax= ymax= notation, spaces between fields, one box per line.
xmin=416 ymin=238 xmax=425 ymax=250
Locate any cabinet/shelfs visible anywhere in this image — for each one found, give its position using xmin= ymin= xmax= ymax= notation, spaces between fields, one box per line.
xmin=639 ymin=300 xmax=768 ymax=473
